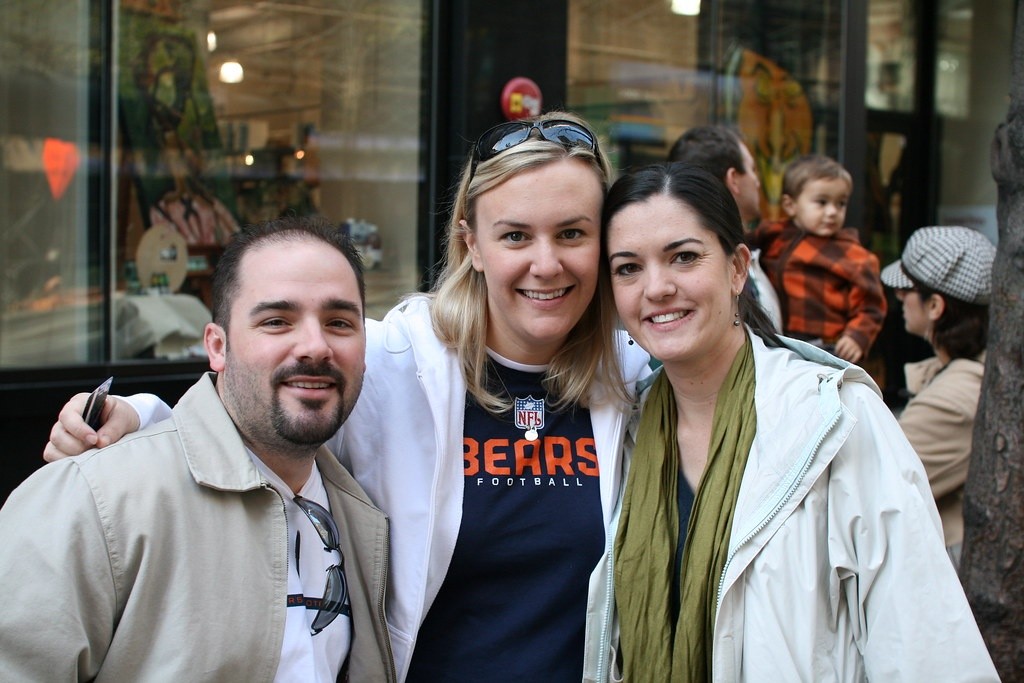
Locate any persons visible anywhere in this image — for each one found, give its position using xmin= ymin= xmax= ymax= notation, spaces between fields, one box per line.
xmin=43 ymin=113 xmax=654 ymax=683
xmin=0 ymin=217 xmax=395 ymax=683
xmin=766 ymin=156 xmax=887 ymax=363
xmin=667 ymin=125 xmax=783 ymax=337
xmin=880 ymin=226 xmax=998 ymax=572
xmin=584 ymin=162 xmax=1001 ymax=683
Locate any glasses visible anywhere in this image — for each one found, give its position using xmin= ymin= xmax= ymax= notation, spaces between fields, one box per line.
xmin=294 ymin=493 xmax=347 ymax=636
xmin=469 ymin=119 xmax=603 ymax=182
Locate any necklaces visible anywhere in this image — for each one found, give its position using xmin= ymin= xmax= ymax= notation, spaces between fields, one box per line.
xmin=491 ymin=359 xmax=550 ymax=441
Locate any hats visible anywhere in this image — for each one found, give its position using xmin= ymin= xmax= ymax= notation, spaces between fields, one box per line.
xmin=879 ymin=225 xmax=998 ymax=306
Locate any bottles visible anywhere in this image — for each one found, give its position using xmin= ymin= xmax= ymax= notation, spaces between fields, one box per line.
xmin=366 ymin=225 xmax=383 ymax=269
